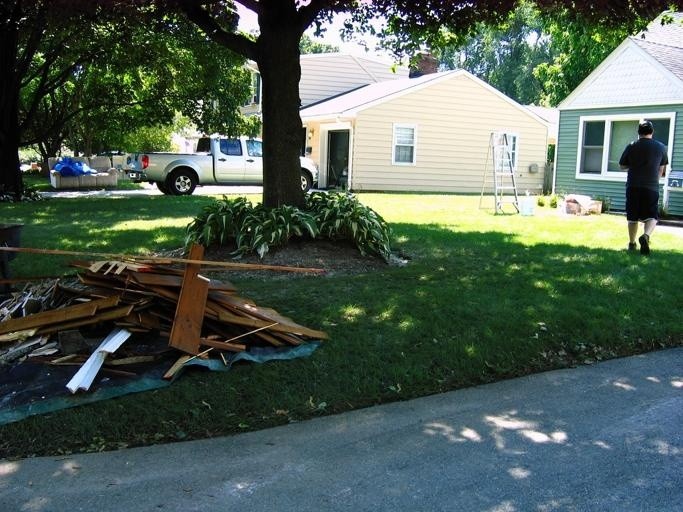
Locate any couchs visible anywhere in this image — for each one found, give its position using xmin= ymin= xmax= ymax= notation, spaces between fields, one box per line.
xmin=47 ymin=156 xmax=118 ymax=189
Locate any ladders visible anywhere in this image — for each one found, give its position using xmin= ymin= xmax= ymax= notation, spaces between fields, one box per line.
xmin=479 ymin=132 xmax=520 ymax=215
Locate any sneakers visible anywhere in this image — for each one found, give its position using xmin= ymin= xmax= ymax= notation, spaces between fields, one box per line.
xmin=640 ymin=233 xmax=650 ymax=254
xmin=628 ymin=242 xmax=636 ymax=250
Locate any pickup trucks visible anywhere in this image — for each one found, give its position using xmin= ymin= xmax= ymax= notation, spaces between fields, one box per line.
xmin=121 ymin=135 xmax=319 ymax=196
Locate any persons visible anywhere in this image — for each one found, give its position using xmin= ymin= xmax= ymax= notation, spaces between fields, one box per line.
xmin=620 ymin=120 xmax=668 ymax=254
